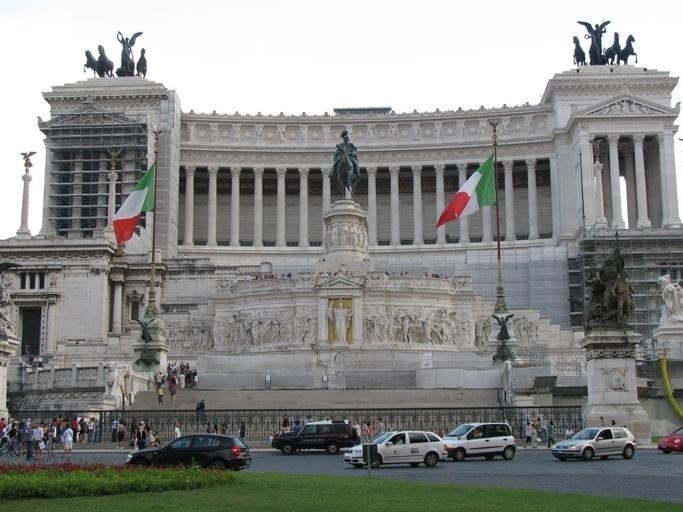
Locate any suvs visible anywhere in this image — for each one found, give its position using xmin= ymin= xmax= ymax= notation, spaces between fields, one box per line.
xmin=443 ymin=422 xmax=516 ymax=460
xmin=272 ymin=420 xmax=362 ymax=456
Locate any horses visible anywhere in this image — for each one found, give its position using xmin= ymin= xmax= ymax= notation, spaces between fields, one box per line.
xmin=136 ymin=48 xmax=147 ymax=79
xmin=604 ymin=32 xmax=622 ymax=66
xmin=96 ymin=44 xmax=113 ymax=78
xmin=83 ymin=50 xmax=96 ymax=77
xmin=572 ymin=36 xmax=585 ymax=67
xmin=616 ymin=34 xmax=638 ymax=65
xmin=334 ymin=142 xmax=357 ymax=200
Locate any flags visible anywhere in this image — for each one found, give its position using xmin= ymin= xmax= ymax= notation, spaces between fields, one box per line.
xmin=113 ymin=160 xmax=157 ymax=245
xmin=436 ymin=151 xmax=498 ymax=227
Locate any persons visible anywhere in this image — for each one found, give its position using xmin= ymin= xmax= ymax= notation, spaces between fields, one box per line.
xmin=305 ymin=414 xmax=311 ymax=425
xmin=566 ymin=427 xmax=574 ymax=440
xmin=476 ymin=317 xmax=495 ymax=346
xmin=333 ymin=130 xmax=360 ymax=190
xmin=352 ymin=418 xmax=384 ymax=440
xmin=282 ymin=414 xmax=290 ymax=433
xmin=293 ymin=420 xmax=302 ymax=434
xmin=175 ymin=422 xmax=180 ymax=439
xmin=24 ymin=342 xmax=31 ymax=355
xmin=424 ymin=271 xmax=448 ymax=279
xmin=164 ymin=322 xmax=215 ymax=351
xmin=221 ymin=313 xmax=314 ymax=345
xmin=330 ymin=300 xmax=352 ymax=341
xmin=240 ymin=420 xmax=245 ymax=440
xmin=29 ymin=354 xmax=34 ymax=366
xmin=383 ymin=270 xmax=408 ymax=277
xmin=207 ymin=420 xmax=229 ymax=434
xmin=323 ymin=417 xmax=332 ymax=422
xmin=1 ymin=415 xmax=159 ymax=464
xmin=155 ymin=360 xmax=205 ymax=410
xmin=251 ymin=272 xmax=292 ymax=279
xmin=515 ymin=316 xmax=540 ymax=338
xmin=523 ymin=416 xmax=557 ymax=449
xmin=600 ymin=416 xmax=607 ymax=427
xmin=317 ymin=271 xmax=337 ymax=276
xmin=365 ymin=308 xmax=456 ymax=345
xmin=612 ymin=420 xmax=617 ymax=427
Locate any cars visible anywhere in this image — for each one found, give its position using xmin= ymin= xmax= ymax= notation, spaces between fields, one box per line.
xmin=551 ymin=427 xmax=637 ymax=460
xmin=344 ymin=430 xmax=448 ymax=467
xmin=657 ymin=428 xmax=682 ymax=454
xmin=125 ymin=434 xmax=251 ymax=471
